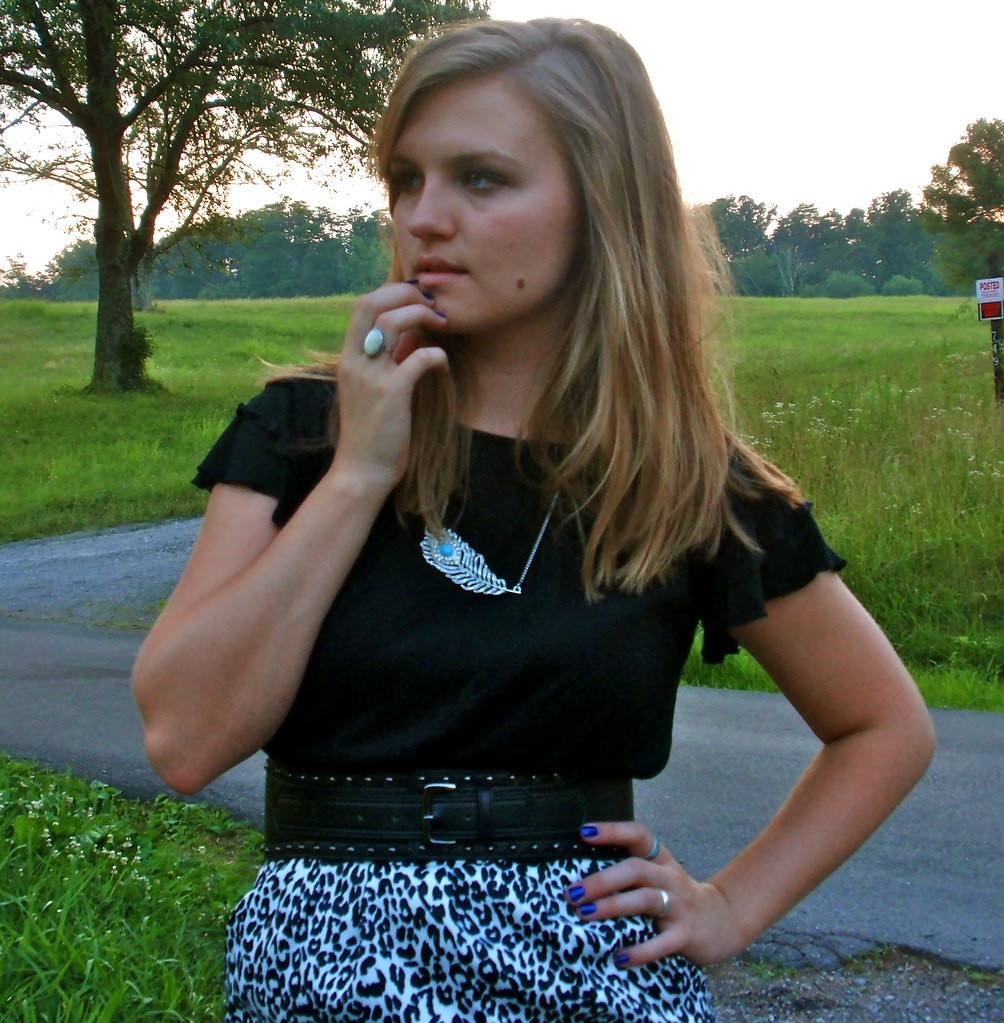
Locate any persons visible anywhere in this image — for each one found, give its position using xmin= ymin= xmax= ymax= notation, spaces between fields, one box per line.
xmin=131 ymin=18 xmax=936 ymax=1023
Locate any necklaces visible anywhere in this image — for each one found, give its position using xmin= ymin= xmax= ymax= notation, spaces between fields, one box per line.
xmin=420 ymin=490 xmax=561 ymax=596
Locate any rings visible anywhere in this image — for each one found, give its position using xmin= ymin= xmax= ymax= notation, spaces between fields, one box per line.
xmin=642 ymin=834 xmax=660 ymax=861
xmin=656 ymin=889 xmax=669 ymax=918
xmin=363 ymin=328 xmax=395 ymax=359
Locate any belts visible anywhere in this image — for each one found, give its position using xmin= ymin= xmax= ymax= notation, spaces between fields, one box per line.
xmin=263 ymin=760 xmax=635 ymax=853
